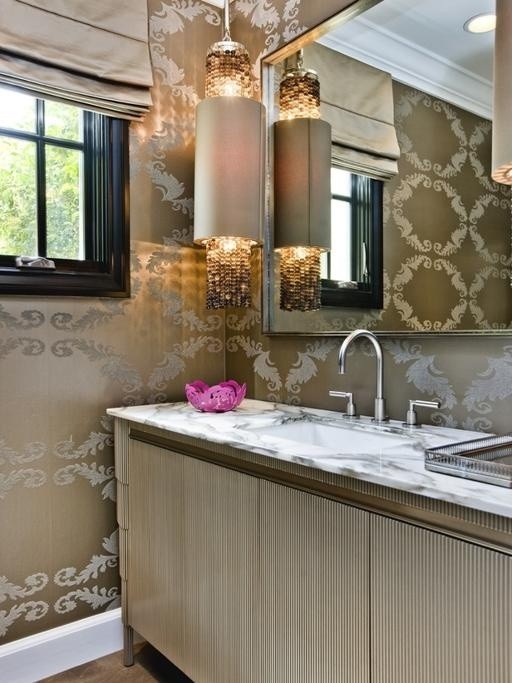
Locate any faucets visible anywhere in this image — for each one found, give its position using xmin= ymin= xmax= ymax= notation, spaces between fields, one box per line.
xmin=337 ymin=327 xmax=385 ymax=421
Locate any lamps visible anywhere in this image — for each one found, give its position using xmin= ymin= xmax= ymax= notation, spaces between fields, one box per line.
xmin=190 ymin=0 xmax=264 ymax=312
xmin=271 ymin=49 xmax=333 ymax=313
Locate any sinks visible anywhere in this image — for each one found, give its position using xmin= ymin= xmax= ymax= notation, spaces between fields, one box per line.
xmin=234 ymin=413 xmax=415 ymax=452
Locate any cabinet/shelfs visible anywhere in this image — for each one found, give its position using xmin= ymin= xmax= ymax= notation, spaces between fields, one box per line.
xmin=120 ymin=432 xmax=258 ymax=683
xmin=257 ymin=454 xmax=510 ymax=682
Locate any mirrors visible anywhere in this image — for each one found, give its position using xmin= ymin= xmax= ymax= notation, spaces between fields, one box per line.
xmin=257 ymin=0 xmax=511 ymax=341
